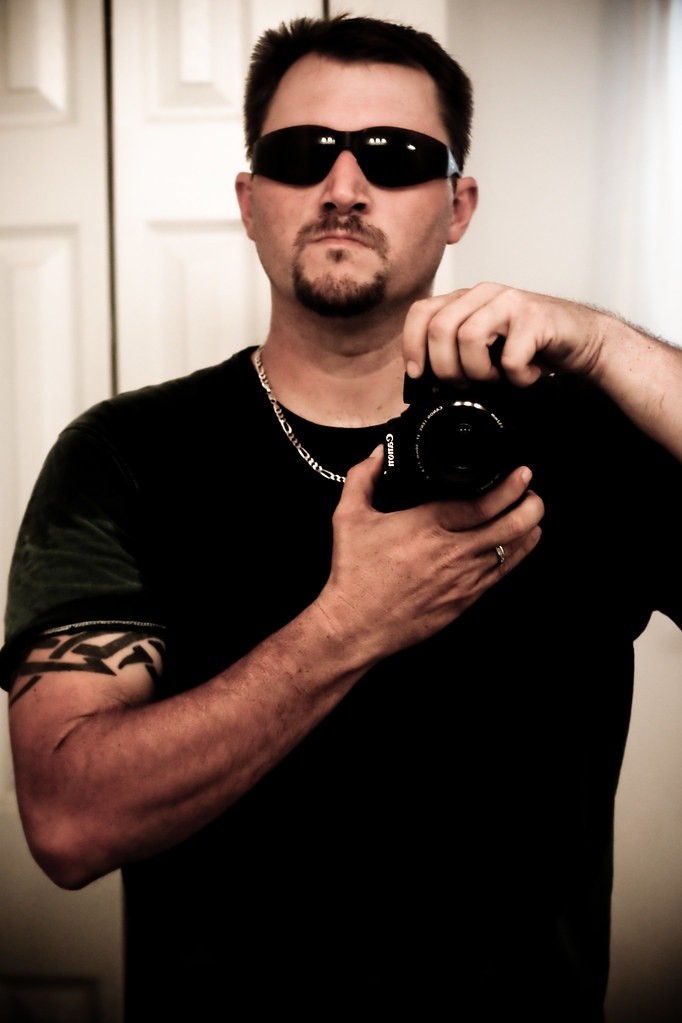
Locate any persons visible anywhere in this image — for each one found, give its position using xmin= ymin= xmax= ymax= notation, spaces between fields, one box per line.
xmin=0 ymin=12 xmax=682 ymax=1023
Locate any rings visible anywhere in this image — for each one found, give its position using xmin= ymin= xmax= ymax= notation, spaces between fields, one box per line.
xmin=496 ymin=546 xmax=505 ymax=563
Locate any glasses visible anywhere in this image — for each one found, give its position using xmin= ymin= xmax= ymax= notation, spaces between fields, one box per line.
xmin=250 ymin=124 xmax=461 ymax=188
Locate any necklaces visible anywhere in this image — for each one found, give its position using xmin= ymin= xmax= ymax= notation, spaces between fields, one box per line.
xmin=256 ymin=345 xmax=386 ymax=484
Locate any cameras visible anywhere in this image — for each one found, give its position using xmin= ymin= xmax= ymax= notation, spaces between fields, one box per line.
xmin=381 ymin=337 xmax=556 ymax=531
